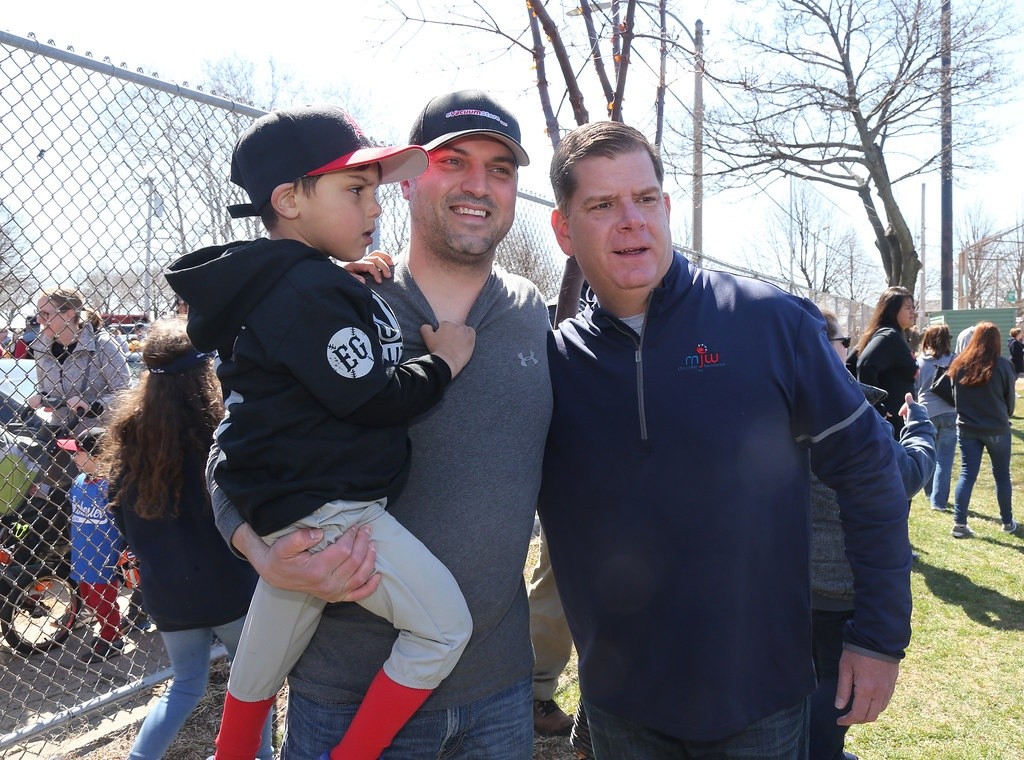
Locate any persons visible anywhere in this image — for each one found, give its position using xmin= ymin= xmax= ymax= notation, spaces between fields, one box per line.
xmin=817 ymin=302 xmax=938 ymax=501
xmin=26 ymin=290 xmax=150 ymax=633
xmin=526 ymin=520 xmax=575 ymax=736
xmin=161 ymin=103 xmax=478 ymax=759
xmin=537 ymin=121 xmax=913 ymax=760
xmin=94 ymin=312 xmax=275 ymax=759
xmin=203 ymin=88 xmax=557 ymax=760
xmin=856 ymin=286 xmax=1024 ymax=537
xmin=57 ymin=426 xmax=128 ymax=664
xmin=0 ymin=316 xmax=41 ymax=359
xmin=107 ymin=322 xmax=147 ymax=353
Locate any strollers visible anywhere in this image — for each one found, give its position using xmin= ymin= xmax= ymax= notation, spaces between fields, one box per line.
xmin=0 ymin=397 xmax=105 ymax=658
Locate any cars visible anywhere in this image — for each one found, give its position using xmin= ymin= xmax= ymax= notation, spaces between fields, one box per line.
xmin=0 ymin=314 xmax=156 ymax=440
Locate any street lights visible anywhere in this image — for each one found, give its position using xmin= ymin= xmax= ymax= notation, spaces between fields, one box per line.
xmin=564 ymin=0 xmax=705 ymax=271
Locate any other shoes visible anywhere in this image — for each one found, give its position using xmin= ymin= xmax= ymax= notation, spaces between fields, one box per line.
xmin=911 ymin=553 xmax=919 ymax=559
xmin=931 ymin=506 xmax=947 ymax=512
xmin=121 ymin=615 xmax=151 ymax=632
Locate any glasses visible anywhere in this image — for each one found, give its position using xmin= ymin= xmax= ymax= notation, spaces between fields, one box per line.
xmin=34 ymin=309 xmax=68 ymax=319
xmin=826 ymin=337 xmax=851 ymax=348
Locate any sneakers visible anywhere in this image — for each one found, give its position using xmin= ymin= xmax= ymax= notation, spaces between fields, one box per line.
xmin=1001 ymin=520 xmax=1017 ymax=534
xmin=50 ymin=607 xmax=98 ymax=630
xmin=950 ymin=525 xmax=975 ymax=537
xmin=533 ymin=698 xmax=574 ymax=737
xmin=90 ymin=637 xmax=98 ymax=646
xmin=81 ymin=639 xmax=125 ymax=663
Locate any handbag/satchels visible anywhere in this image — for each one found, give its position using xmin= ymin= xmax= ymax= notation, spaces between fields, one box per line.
xmin=928 ymin=352 xmax=955 ymax=408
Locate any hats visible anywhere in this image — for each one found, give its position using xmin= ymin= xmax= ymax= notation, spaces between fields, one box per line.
xmin=226 ymin=106 xmax=430 ymax=219
xmin=408 ymin=90 xmax=530 ymax=166
xmin=56 ymin=427 xmax=111 ymax=454
